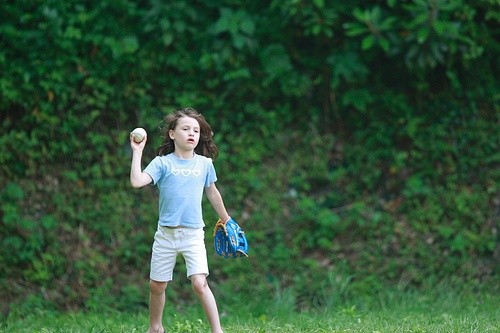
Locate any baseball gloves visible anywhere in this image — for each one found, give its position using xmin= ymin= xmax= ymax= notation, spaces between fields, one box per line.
xmin=213 ymin=216 xmax=248 ymax=258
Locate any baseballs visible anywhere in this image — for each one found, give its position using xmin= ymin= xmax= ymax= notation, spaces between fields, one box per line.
xmin=132 ymin=128 xmax=146 ymax=143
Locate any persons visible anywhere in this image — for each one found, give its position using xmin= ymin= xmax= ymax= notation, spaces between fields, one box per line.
xmin=128 ymin=107 xmax=235 ymax=333
